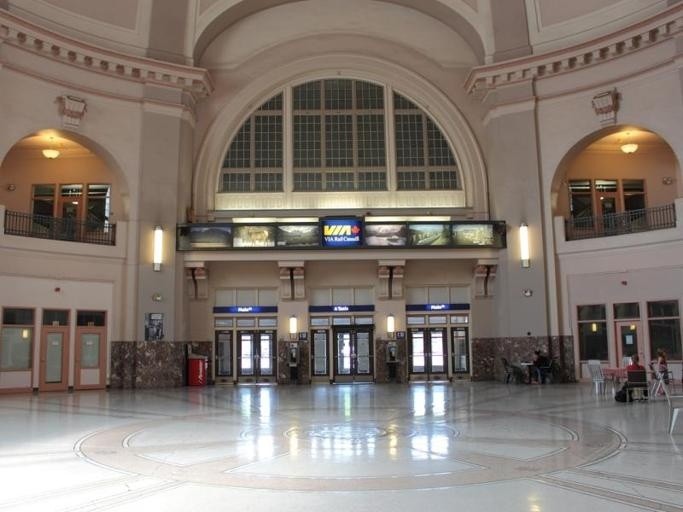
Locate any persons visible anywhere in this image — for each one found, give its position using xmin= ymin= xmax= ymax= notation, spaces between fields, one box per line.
xmin=657 ymin=350 xmax=669 ymax=395
xmin=510 ymin=352 xmax=529 ymax=384
xmin=626 ymin=354 xmax=648 ymax=400
xmin=532 ymin=350 xmax=548 ymax=384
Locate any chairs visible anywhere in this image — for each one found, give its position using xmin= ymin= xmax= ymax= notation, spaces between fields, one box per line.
xmin=501 ymin=357 xmax=554 ymax=384
xmin=585 ymin=357 xmax=683 ymax=437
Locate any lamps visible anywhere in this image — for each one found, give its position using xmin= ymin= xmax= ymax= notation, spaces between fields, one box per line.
xmin=619 ymin=133 xmax=639 ymax=155
xmin=41 ymin=137 xmax=60 ymax=160
xmin=152 ymin=224 xmax=164 ymax=273
xmin=288 ymin=313 xmax=297 ymax=340
xmin=518 ymin=220 xmax=530 ymax=269
xmin=386 ymin=311 xmax=395 ymax=338
